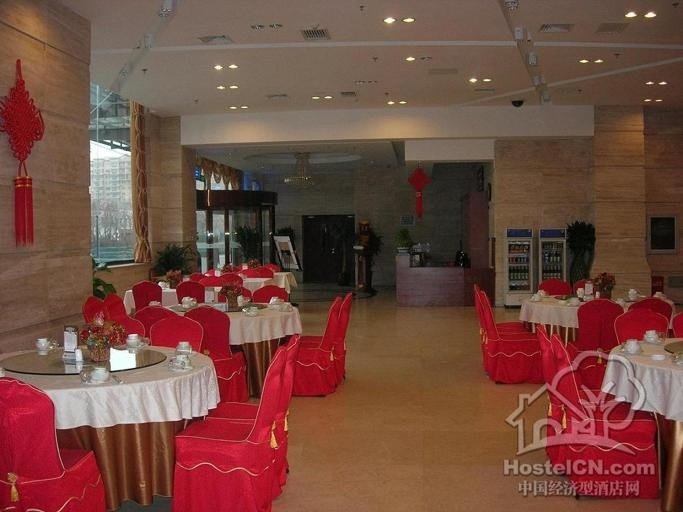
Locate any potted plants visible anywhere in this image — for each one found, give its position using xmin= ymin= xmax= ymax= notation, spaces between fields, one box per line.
xmin=564 ymin=220 xmax=597 ymax=295
xmin=392 ymin=227 xmax=413 ymax=253
xmin=355 ymin=229 xmax=384 ymax=295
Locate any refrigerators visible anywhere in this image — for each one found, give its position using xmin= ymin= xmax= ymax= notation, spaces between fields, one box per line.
xmin=538 ymin=227 xmax=566 ymax=283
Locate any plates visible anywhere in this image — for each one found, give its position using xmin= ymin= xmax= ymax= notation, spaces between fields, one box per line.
xmin=91 ymin=371 xmax=109 ymax=380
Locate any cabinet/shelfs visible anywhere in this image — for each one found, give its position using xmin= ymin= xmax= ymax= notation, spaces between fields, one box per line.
xmin=538 ymin=226 xmax=570 ymax=296
xmin=456 ymin=190 xmax=492 ymax=306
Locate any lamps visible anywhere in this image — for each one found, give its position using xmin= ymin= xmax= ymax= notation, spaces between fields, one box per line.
xmin=496 ymin=0 xmax=558 ymax=105
xmin=281 ymin=152 xmax=319 ymax=190
xmin=156 ymin=1 xmax=177 ymax=19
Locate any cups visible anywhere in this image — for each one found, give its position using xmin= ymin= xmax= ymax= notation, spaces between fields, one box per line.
xmin=38 ymin=350 xmax=47 ymax=355
xmin=93 ymin=367 xmax=106 ymax=374
xmin=127 ymin=334 xmax=138 ymax=340
xmin=35 ymin=338 xmax=48 ymax=344
xmin=177 ymin=341 xmax=189 ymax=347
xmin=175 ymin=355 xmax=187 ymax=361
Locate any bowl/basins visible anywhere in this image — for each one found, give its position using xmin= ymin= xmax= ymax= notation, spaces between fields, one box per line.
xmin=175 ymin=358 xmax=190 ymax=366
xmin=175 ymin=347 xmax=192 ymax=355
xmin=34 ymin=343 xmax=49 ymax=350
xmin=147 ymin=295 xmax=293 ymax=316
xmin=530 ymin=288 xmax=662 ymax=308
xmin=125 ymin=339 xmax=140 ymax=346
xmin=623 ymin=329 xmax=659 ymax=353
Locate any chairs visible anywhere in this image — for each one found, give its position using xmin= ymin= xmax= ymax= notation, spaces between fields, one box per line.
xmin=470 ymin=272 xmax=683 ymax=512
xmin=0 ymin=257 xmax=354 ymax=511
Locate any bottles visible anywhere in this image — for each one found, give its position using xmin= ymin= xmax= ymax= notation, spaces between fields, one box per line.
xmin=74 ymin=346 xmax=83 ymax=362
xmin=75 ymin=361 xmax=82 ymax=372
xmin=508 ymin=245 xmax=530 ymax=290
xmin=543 ymin=255 xmax=561 ymax=281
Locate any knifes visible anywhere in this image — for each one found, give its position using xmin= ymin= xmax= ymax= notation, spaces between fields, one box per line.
xmin=111 ymin=374 xmax=122 ymax=384
